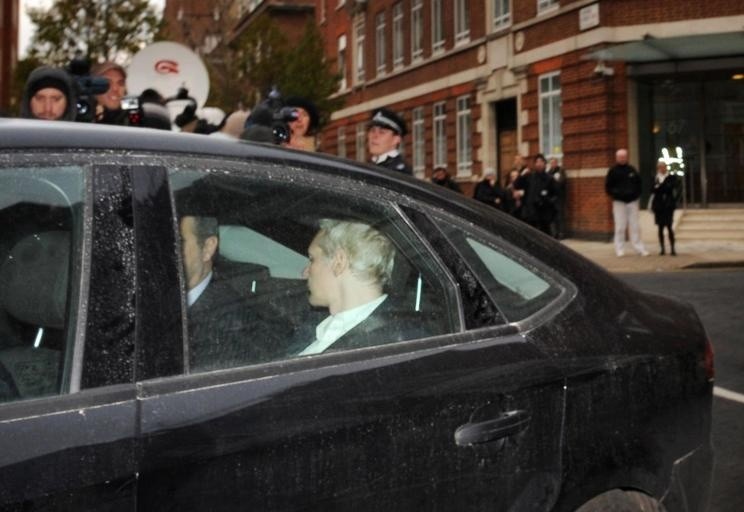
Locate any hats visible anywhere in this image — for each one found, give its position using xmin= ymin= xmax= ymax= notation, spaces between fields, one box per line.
xmin=364 ymin=106 xmax=411 ymax=138
xmin=93 ymin=61 xmax=128 ymax=80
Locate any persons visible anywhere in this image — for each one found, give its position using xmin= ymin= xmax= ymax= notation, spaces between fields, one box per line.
xmin=171 ymin=213 xmax=286 ymax=374
xmin=501 ymin=153 xmax=567 ymax=241
xmin=604 ymin=148 xmax=650 ymax=258
xmin=284 ymin=218 xmax=433 ymax=362
xmin=471 ymin=167 xmax=505 ymax=210
xmin=429 ymin=163 xmax=463 ymax=194
xmin=206 ymin=108 xmax=251 ymax=139
xmin=84 ymin=61 xmax=132 ymax=127
xmin=17 ymin=63 xmax=77 ymax=123
xmin=275 ymin=95 xmax=321 ymax=153
xmin=647 ymin=161 xmax=682 ymax=256
xmin=138 ymin=89 xmax=199 ymax=133
xmin=361 ymin=107 xmax=409 ymax=174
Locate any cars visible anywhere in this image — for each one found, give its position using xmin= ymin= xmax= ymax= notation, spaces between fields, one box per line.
xmin=2 ymin=117 xmax=716 ymax=506
